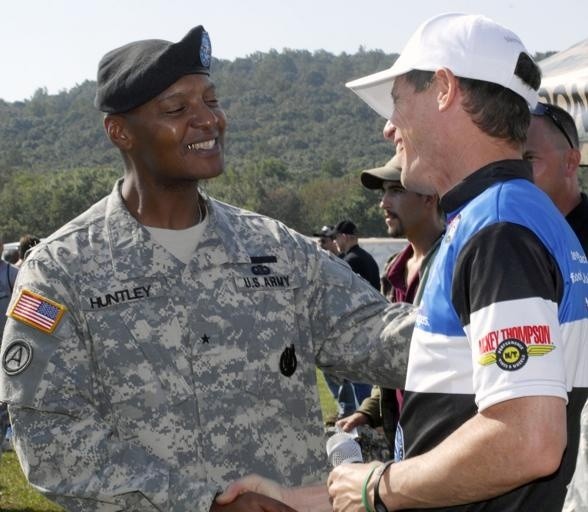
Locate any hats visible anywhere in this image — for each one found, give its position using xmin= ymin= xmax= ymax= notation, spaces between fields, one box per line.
xmin=94 ymin=26 xmax=211 ymax=114
xmin=361 ymin=153 xmax=402 ymax=190
xmin=345 ymin=13 xmax=541 ymax=121
xmin=314 ymin=225 xmax=332 ymax=237
xmin=325 ymin=220 xmax=359 ymax=237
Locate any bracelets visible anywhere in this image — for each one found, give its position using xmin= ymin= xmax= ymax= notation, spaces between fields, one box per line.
xmin=374 ymin=460 xmax=398 ymax=512
xmin=360 ymin=460 xmax=382 ymax=512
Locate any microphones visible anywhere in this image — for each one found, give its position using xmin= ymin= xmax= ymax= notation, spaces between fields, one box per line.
xmin=326 ymin=432 xmax=364 ymax=469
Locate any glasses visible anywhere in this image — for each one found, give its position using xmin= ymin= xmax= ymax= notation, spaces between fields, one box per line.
xmin=530 ymin=103 xmax=574 ymax=150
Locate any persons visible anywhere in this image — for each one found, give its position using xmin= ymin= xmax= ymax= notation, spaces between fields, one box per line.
xmin=0 ymin=233 xmax=40 ymax=452
xmin=525 ymin=103 xmax=587 ymax=257
xmin=331 ymin=151 xmax=445 ymax=461
xmin=0 ymin=22 xmax=418 ymax=511
xmin=330 ymin=220 xmax=382 ymax=412
xmin=208 ymin=10 xmax=586 ymax=511
xmin=312 ymin=222 xmax=341 ymax=402
